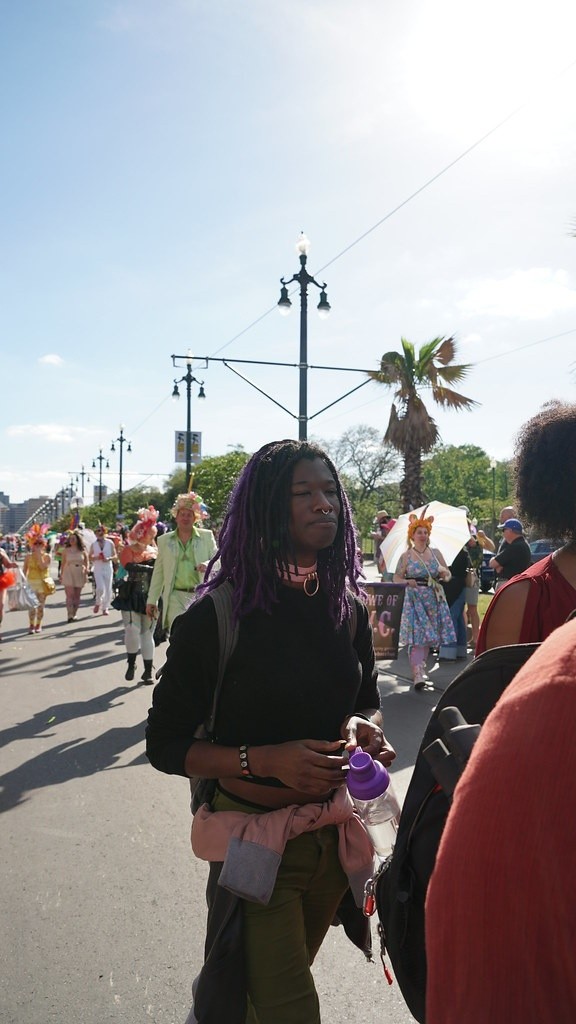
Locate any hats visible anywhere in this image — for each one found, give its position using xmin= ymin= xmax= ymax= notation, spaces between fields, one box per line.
xmin=497 ymin=519 xmax=522 ymax=531
xmin=408 ymin=514 xmax=434 ymax=539
xmin=458 ymin=506 xmax=469 ymax=514
xmin=373 ymin=510 xmax=390 ymax=524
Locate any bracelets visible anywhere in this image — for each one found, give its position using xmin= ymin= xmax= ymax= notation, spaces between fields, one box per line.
xmin=347 ymin=713 xmax=371 ymax=722
xmin=239 ymin=744 xmax=254 ymax=778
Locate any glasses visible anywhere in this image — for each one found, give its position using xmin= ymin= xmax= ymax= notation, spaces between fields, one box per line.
xmin=502 ymin=528 xmax=508 ymax=533
xmin=33 ymin=542 xmax=42 ymax=546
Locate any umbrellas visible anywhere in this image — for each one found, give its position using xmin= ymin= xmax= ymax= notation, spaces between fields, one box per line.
xmin=379 ymin=500 xmax=471 ymax=574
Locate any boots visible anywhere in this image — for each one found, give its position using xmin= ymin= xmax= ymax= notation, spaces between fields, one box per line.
xmin=141 ymin=659 xmax=154 ymax=684
xmin=125 ymin=652 xmax=138 ymax=680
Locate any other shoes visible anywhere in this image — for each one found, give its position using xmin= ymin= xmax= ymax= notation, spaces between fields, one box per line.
xmin=68 ymin=618 xmax=74 ymax=623
xmin=103 ymin=610 xmax=108 ymax=615
xmin=35 ymin=626 xmax=41 ymax=632
xmin=435 ymin=657 xmax=455 ymax=664
xmin=466 ymin=640 xmax=476 ymax=648
xmin=414 ymin=670 xmax=425 ymax=689
xmin=29 ymin=629 xmax=35 ymax=633
xmin=455 ymin=656 xmax=467 ymax=660
xmin=94 ymin=605 xmax=99 ymax=613
xmin=419 ymin=664 xmax=429 ymax=680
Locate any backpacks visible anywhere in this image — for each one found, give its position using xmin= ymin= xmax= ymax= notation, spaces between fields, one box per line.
xmin=361 ymin=607 xmax=576 ymax=1024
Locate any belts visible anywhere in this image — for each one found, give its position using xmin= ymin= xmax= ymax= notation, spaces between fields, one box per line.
xmin=176 ymin=588 xmax=197 ymax=593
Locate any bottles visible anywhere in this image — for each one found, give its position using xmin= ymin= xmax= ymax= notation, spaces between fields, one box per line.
xmin=345 ymin=745 xmax=401 ymax=864
xmin=149 ymin=608 xmax=157 ymax=633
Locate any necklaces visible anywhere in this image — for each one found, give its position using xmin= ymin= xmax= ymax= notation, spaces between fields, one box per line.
xmin=276 ymin=559 xmax=319 ymax=596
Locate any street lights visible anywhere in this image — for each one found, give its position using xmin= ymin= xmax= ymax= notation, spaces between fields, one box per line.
xmin=15 ymin=443 xmax=112 ymax=540
xmin=275 ymin=229 xmax=332 ymax=442
xmin=170 ymin=345 xmax=206 ymax=488
xmin=108 ymin=421 xmax=132 ymax=515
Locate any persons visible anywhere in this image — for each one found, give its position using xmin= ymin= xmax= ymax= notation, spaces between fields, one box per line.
xmin=146 ymin=492 xmax=222 ymax=635
xmin=110 ymin=506 xmax=159 ymax=685
xmin=1 ymin=536 xmax=22 ymax=559
xmin=54 ymin=533 xmax=65 ymax=578
xmin=88 ymin=525 xmax=118 ymax=615
xmin=145 ymin=439 xmax=396 ymax=1024
xmin=424 ymin=616 xmax=576 ymax=1024
xmin=59 ymin=528 xmax=90 ymax=622
xmin=368 ymin=510 xmax=394 ymax=582
xmin=392 ymin=516 xmax=458 ymax=688
xmin=431 ymin=506 xmax=532 ymax=661
xmin=474 ymin=404 xmax=576 ymax=657
xmin=21 ymin=524 xmax=56 ymax=634
xmin=0 ymin=545 xmax=19 ymax=623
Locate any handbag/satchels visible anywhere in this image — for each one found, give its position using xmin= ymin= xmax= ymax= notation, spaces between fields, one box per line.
xmin=465 ymin=568 xmax=476 ymax=588
xmin=427 ymin=577 xmax=446 ymax=602
xmin=42 ymin=577 xmax=55 ymax=594
xmin=7 ymin=567 xmax=40 ymax=611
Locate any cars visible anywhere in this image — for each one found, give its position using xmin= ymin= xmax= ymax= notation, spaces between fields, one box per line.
xmin=528 ymin=538 xmax=567 ymax=568
xmin=478 ymin=549 xmax=499 ymax=593
